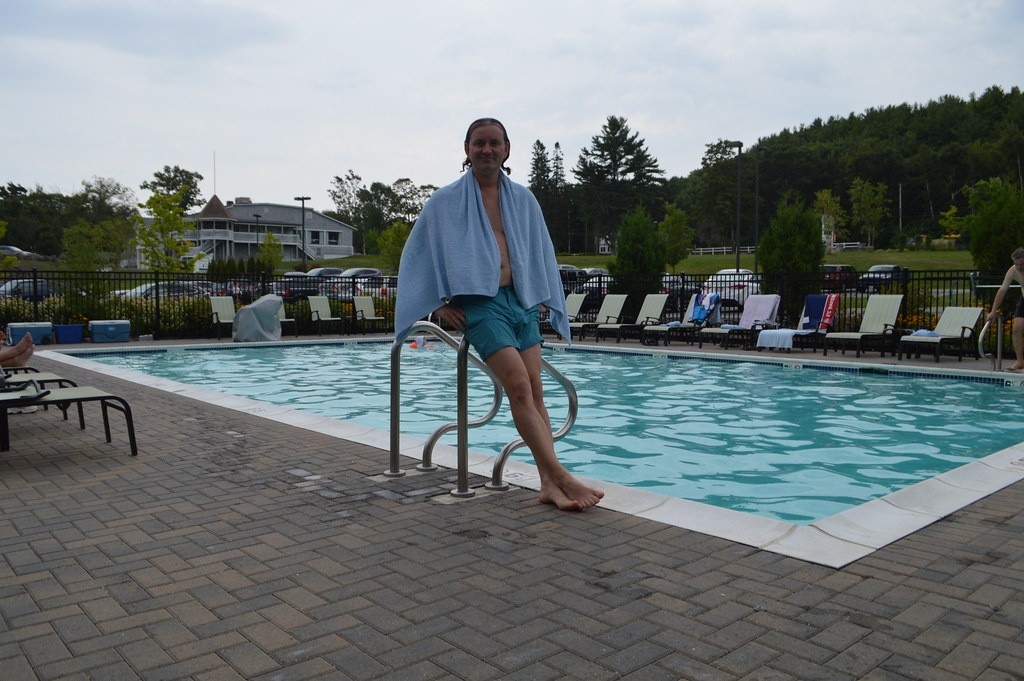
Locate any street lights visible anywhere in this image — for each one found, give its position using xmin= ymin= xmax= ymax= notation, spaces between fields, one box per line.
xmin=253 ymin=214 xmax=262 ymax=282
xmin=754 ymin=145 xmax=768 ymax=274
xmin=293 ymin=196 xmax=312 ymax=264
xmin=725 ymin=141 xmax=743 ymax=273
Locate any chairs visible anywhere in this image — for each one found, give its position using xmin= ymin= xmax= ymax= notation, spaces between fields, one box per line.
xmin=0 ymin=365 xmax=137 ymax=456
xmin=699 ymin=294 xmax=781 ymax=349
xmin=641 ymin=293 xmax=722 ymax=346
xmin=898 ymin=307 xmax=984 ymax=362
xmin=307 ymin=296 xmax=343 ymax=336
xmin=595 ymin=294 xmax=671 ymax=343
xmin=351 ymin=296 xmax=389 ymax=335
xmin=558 ymin=294 xmax=629 ymax=341
xmin=205 ymin=295 xmax=237 ymax=341
xmin=539 ymin=294 xmax=588 ymax=340
xmin=758 ymin=294 xmax=840 ymax=355
xmin=823 ymin=295 xmax=903 ymax=358
xmin=278 ymin=296 xmax=298 ymax=338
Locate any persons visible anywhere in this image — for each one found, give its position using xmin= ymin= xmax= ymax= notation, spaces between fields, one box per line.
xmin=394 ymin=118 xmax=605 ymax=511
xmin=416 ymin=336 xmax=427 ymax=346
xmin=0 ymin=332 xmax=35 ymax=367
xmin=987 ymin=247 xmax=1024 ymax=371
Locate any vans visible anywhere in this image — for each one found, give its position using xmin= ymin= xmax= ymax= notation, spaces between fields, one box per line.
xmin=0 ymin=277 xmax=48 ymax=307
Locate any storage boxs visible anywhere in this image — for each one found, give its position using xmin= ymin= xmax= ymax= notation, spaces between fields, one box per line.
xmin=6 ymin=322 xmax=53 ymax=346
xmin=54 ymin=324 xmax=86 ymax=345
xmin=88 ymin=320 xmax=131 ymax=343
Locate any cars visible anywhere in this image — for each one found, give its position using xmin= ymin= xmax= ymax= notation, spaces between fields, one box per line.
xmin=816 ymin=263 xmax=861 ymax=293
xmin=858 ymin=263 xmax=904 ymax=294
xmin=556 ymin=262 xmax=762 ymax=317
xmin=0 ymin=245 xmax=27 ymax=259
xmin=110 ymin=266 xmax=398 ymax=308
